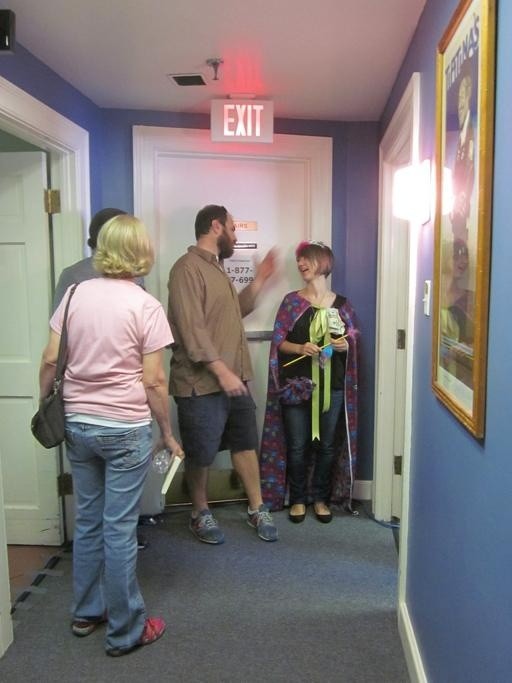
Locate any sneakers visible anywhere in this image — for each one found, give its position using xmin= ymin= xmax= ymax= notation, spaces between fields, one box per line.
xmin=103 ymin=616 xmax=165 ymax=657
xmin=247 ymin=503 xmax=280 ymax=542
xmin=188 ymin=509 xmax=225 ymax=545
xmin=72 ymin=607 xmax=108 ymax=637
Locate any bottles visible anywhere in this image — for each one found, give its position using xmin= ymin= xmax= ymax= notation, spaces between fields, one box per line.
xmin=151 ymin=448 xmax=172 ymax=475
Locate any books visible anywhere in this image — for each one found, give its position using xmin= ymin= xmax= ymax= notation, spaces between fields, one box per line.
xmin=160 ymin=456 xmax=181 ymax=495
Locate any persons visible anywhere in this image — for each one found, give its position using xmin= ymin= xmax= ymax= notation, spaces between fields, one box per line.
xmin=167 ymin=203 xmax=285 ymax=544
xmin=258 ymin=238 xmax=364 ymax=525
xmin=50 ymin=207 xmax=161 ymax=553
xmin=442 ymin=66 xmax=478 ymax=339
xmin=39 ymin=212 xmax=185 ymax=658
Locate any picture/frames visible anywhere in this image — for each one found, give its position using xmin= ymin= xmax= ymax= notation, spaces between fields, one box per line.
xmin=427 ymin=0 xmax=498 ymax=442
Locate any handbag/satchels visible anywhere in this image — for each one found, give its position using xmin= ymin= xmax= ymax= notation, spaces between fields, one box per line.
xmin=30 ymin=387 xmax=65 ymax=450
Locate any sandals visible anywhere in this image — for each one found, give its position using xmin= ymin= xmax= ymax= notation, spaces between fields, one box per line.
xmin=313 ymin=503 xmax=332 ymax=523
xmin=289 ymin=504 xmax=307 ymax=523
